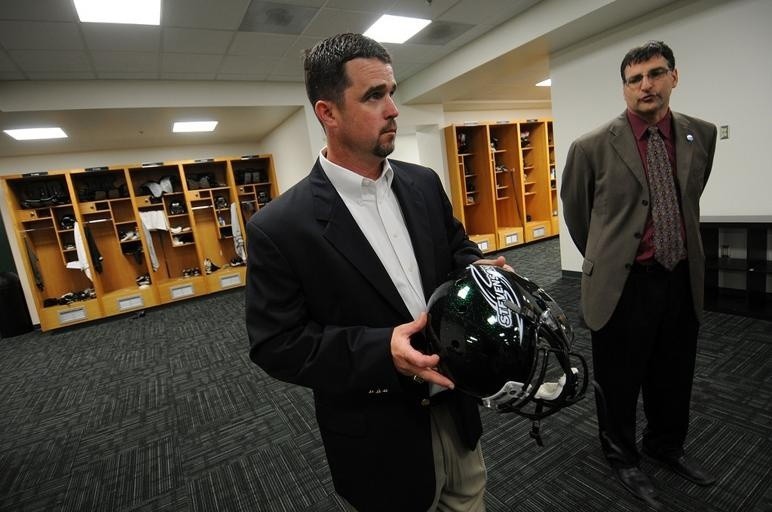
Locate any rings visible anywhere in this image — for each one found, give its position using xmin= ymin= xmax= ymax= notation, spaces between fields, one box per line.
xmin=412 ymin=374 xmax=425 ymax=383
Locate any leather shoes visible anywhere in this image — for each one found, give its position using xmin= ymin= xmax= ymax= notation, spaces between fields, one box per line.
xmin=616 ymin=465 xmax=656 ymax=498
xmin=668 ymin=454 xmax=718 ymax=486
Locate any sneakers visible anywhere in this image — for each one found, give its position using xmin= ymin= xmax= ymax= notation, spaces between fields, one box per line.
xmin=183 ymin=268 xmax=191 ymax=278
xmin=193 ymin=268 xmax=200 ymax=277
xmin=230 ymin=257 xmax=243 ymax=267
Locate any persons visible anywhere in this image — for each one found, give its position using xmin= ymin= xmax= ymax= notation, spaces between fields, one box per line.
xmin=242 ymin=30 xmax=518 ymax=512
xmin=560 ymin=40 xmax=718 ymax=502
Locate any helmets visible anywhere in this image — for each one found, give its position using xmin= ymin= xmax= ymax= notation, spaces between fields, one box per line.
xmin=60 ymin=215 xmax=75 ymax=230
xmin=421 ymin=262 xmax=587 ymax=419
xmin=215 ymin=195 xmax=227 ymax=208
xmin=169 ymin=201 xmax=184 ymax=214
xmin=259 ymin=191 xmax=269 ymax=203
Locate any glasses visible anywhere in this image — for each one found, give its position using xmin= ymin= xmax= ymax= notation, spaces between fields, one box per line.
xmin=625 ymin=67 xmax=670 ymax=89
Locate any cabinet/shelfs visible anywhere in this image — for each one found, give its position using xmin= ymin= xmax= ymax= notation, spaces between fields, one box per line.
xmin=124 ymin=161 xmax=209 ymax=304
xmin=699 ymin=215 xmax=772 ymax=319
xmin=517 ymin=119 xmax=551 ymax=243
xmin=487 ymin=119 xmax=524 ymax=251
xmin=68 ymin=162 xmax=162 ymax=318
xmin=543 ymin=119 xmax=562 ymax=237
xmin=0 ymin=171 xmax=106 ymax=334
xmin=444 ymin=122 xmax=497 ymax=256
xmin=228 ymin=154 xmax=279 ymax=221
xmin=180 ymin=157 xmax=247 ymax=294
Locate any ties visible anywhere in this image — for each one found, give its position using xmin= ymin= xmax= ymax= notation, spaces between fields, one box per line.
xmin=646 ymin=126 xmax=687 ymax=271
